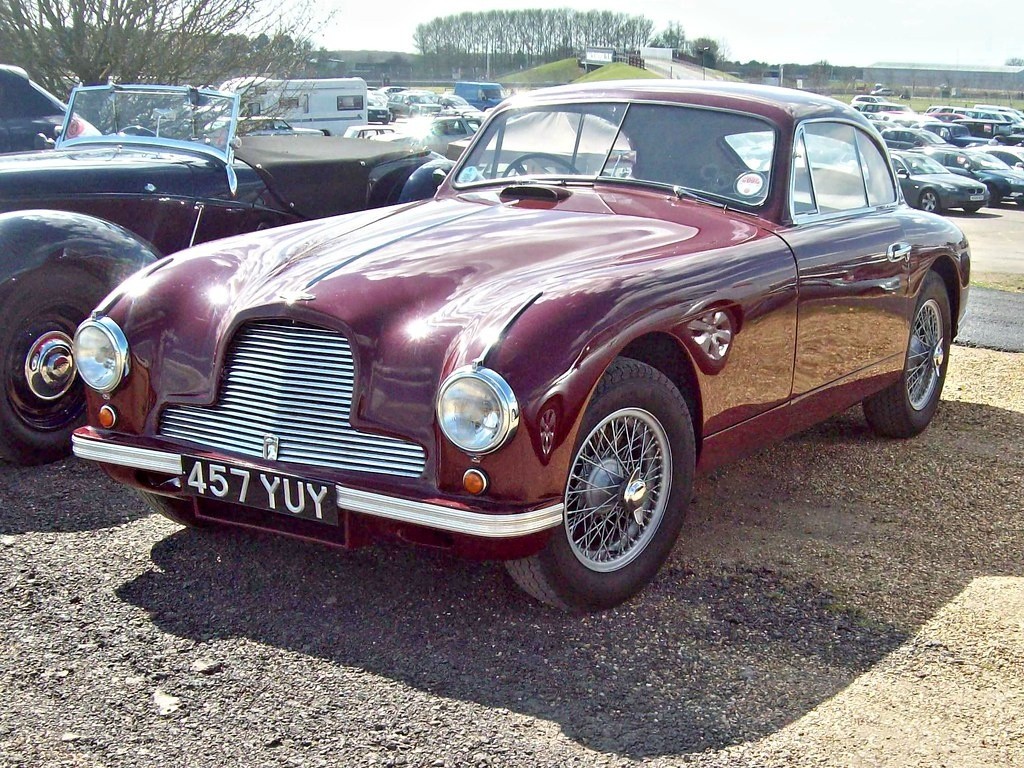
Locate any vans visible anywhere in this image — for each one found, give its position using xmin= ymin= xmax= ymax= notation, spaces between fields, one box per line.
xmin=453 ymin=81 xmax=510 ymax=112
xmin=212 ymin=77 xmax=369 ymax=140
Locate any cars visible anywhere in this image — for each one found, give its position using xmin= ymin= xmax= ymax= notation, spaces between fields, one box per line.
xmin=69 ymin=72 xmax=972 ymax=612
xmin=0 ymin=64 xmax=1024 ymax=469
